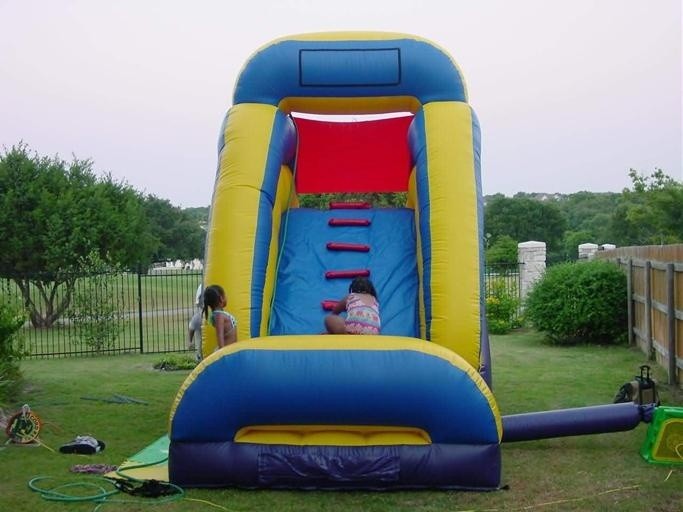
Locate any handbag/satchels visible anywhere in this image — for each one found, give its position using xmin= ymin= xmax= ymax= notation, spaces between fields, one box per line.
xmin=59 ymin=440 xmax=105 ymax=454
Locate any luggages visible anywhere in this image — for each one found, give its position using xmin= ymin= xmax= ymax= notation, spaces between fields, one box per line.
xmin=615 ymin=365 xmax=660 ymax=406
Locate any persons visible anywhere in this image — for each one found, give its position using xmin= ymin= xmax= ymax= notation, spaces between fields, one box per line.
xmin=323 ymin=277 xmax=381 ymax=336
xmin=186 ymin=310 xmax=201 ymax=359
xmin=198 ymin=285 xmax=237 ymax=351
